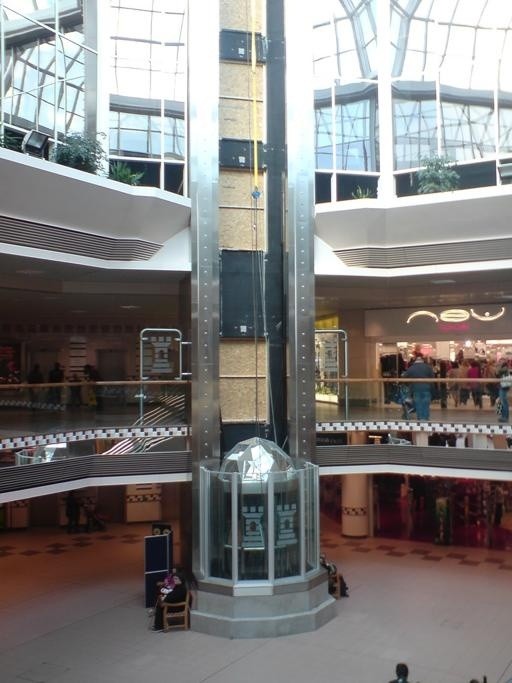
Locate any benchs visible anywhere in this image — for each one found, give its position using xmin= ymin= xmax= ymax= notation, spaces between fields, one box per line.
xmin=156 ymin=582 xmax=191 ymax=634
xmin=325 ymin=568 xmax=347 ymax=602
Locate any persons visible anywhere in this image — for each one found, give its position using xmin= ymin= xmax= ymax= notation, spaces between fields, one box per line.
xmin=392 ymin=347 xmax=512 ymax=425
xmin=389 ymin=663 xmax=410 ymax=683
xmin=320 ymin=552 xmax=347 ymax=598
xmin=146 ymin=567 xmax=196 ymax=632
xmin=0 ymin=356 xmax=108 ymax=419
xmin=66 ymin=493 xmax=85 ymax=532
